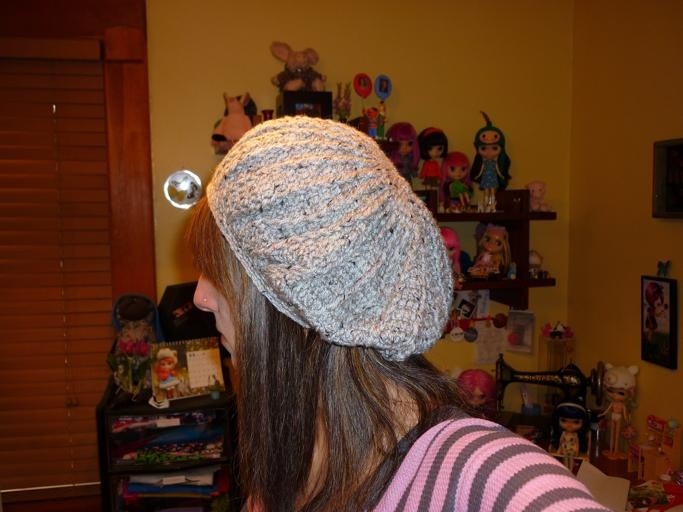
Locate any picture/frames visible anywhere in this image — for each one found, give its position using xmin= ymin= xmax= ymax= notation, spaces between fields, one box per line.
xmin=635 ymin=273 xmax=679 ymax=373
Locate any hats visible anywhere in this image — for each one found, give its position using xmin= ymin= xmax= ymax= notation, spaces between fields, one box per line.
xmin=206 ymin=116 xmax=454 ymax=362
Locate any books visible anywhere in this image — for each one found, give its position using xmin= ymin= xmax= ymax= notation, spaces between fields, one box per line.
xmin=128 ymin=468 xmax=215 ymax=487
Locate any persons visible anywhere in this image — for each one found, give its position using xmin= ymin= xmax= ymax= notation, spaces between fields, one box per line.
xmin=185 ymin=115 xmax=612 ymax=512
xmin=598 ymin=363 xmax=639 ymax=455
xmin=387 ymin=111 xmax=510 ymax=213
xmin=459 ymin=369 xmax=496 ymax=418
xmin=440 ymin=224 xmax=511 ymax=285
xmin=208 ymin=37 xmax=683 ymax=512
xmin=556 ymin=396 xmax=589 ymax=472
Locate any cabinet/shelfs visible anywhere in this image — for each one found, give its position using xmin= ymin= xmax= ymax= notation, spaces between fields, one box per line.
xmin=95 ymin=384 xmax=241 ymax=511
xmin=433 ymin=189 xmax=561 ymax=307
